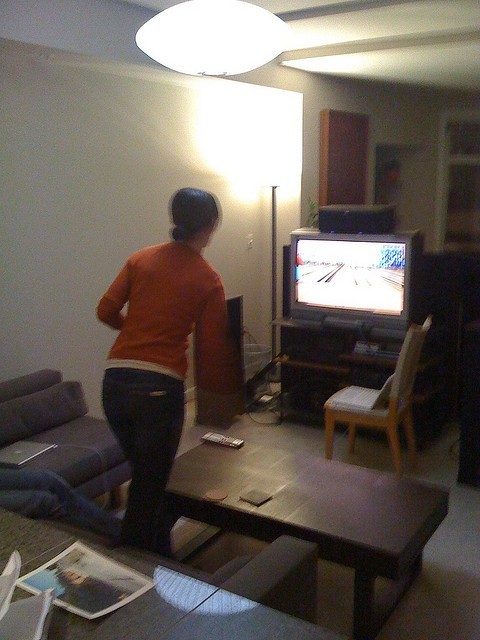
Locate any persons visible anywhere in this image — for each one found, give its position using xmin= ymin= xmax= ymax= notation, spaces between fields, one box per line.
xmin=93 ymin=186 xmax=238 ymax=548
xmin=0 ymin=467 xmax=130 ymax=540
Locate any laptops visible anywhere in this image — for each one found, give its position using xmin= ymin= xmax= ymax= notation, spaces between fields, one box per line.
xmin=332 ymin=372 xmax=396 ymax=411
xmin=0 ymin=439 xmax=58 ymax=469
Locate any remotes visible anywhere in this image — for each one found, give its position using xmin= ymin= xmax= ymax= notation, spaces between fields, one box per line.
xmin=200 ymin=432 xmax=247 ymax=451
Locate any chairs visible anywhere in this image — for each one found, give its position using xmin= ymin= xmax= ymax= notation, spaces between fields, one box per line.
xmin=324 ymin=317 xmax=434 ymax=476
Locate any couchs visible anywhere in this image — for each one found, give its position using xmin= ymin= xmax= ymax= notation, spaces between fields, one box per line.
xmin=0 ymin=369 xmax=133 ymax=508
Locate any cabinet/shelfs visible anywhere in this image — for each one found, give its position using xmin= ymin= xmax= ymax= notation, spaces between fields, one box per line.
xmin=271 ymin=316 xmax=454 ymax=449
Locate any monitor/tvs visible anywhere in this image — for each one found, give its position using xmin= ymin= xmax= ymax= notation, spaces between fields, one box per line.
xmin=288 ymin=228 xmax=425 ymax=342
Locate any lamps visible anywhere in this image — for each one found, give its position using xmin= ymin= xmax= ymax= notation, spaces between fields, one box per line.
xmin=135 ymin=1 xmax=296 ymax=76
